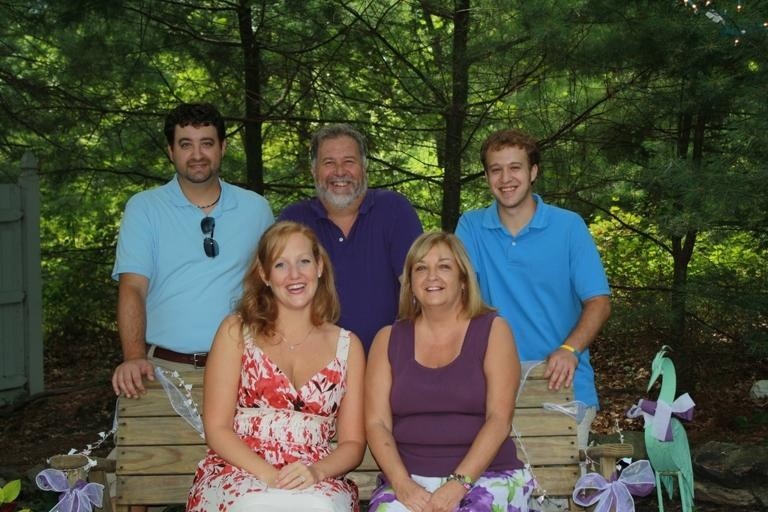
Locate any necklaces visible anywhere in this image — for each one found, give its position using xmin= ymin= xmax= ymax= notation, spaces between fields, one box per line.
xmin=275 ymin=323 xmax=315 ymax=352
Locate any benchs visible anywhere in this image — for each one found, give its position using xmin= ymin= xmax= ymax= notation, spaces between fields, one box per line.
xmin=115 ymin=360 xmax=634 ymax=512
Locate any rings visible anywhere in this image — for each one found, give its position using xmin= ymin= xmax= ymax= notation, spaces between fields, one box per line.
xmin=299 ymin=474 xmax=306 ymax=484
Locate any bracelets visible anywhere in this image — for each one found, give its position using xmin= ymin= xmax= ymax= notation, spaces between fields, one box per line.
xmin=559 ymin=342 xmax=583 ymax=366
xmin=446 ymin=469 xmax=475 ymax=493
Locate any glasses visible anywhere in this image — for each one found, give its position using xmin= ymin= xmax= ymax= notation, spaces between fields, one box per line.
xmin=200 ymin=214 xmax=219 ymax=259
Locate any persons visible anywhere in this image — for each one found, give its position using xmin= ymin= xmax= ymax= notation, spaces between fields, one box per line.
xmin=275 ymin=124 xmax=423 ymax=364
xmin=453 ymin=128 xmax=613 ymax=476
xmin=111 ymin=102 xmax=276 ymax=400
xmin=363 ymin=230 xmax=538 ymax=512
xmin=185 ymin=220 xmax=367 ymax=512
